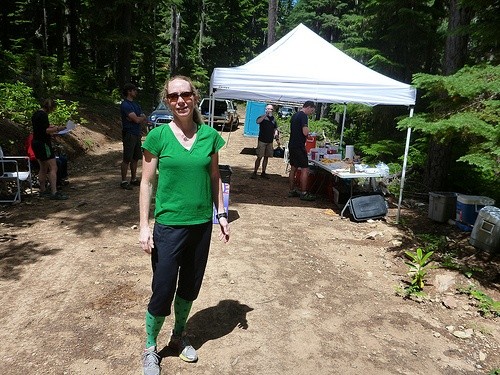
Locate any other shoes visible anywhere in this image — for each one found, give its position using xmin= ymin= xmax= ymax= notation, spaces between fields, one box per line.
xmin=288 ymin=190 xmax=300 ymax=197
xmin=261 ymin=173 xmax=266 ymax=177
xmin=130 ymin=180 xmax=140 ymax=185
xmin=300 ymin=192 xmax=316 ymax=201
xmin=39 ymin=189 xmax=50 ymax=198
xmin=49 ymin=192 xmax=68 ymax=200
xmin=121 ymin=182 xmax=132 ymax=190
xmin=252 ymin=174 xmax=256 ymax=179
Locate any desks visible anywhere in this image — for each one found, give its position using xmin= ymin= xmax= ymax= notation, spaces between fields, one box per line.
xmin=311 ymin=160 xmax=387 ymax=219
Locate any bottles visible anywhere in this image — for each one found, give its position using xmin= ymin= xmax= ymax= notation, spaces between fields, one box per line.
xmin=350 ymin=163 xmax=355 ymax=174
xmin=269 ymin=112 xmax=272 ymax=116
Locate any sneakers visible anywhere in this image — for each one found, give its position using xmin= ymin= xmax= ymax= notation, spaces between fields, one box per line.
xmin=169 ymin=329 xmax=198 ymax=361
xmin=142 ymin=345 xmax=161 ymax=375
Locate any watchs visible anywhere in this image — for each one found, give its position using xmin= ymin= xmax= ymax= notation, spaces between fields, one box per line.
xmin=216 ymin=213 xmax=227 ymax=219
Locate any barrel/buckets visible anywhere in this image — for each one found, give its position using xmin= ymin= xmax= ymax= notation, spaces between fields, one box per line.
xmin=305 ymin=136 xmax=316 ymax=153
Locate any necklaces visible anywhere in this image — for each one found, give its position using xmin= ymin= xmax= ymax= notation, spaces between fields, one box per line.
xmin=180 ymin=135 xmax=190 ymax=141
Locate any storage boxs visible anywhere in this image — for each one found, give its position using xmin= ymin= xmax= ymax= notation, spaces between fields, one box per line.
xmin=428 ymin=191 xmax=461 ymax=223
xmin=456 ymin=195 xmax=500 ymax=254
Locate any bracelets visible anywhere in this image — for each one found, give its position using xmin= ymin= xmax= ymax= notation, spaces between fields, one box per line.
xmin=56 ymin=127 xmax=60 ymax=132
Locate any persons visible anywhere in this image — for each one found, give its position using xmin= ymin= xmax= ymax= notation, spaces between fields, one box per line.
xmin=118 ymin=82 xmax=148 ymax=191
xmin=25 ymin=129 xmax=70 ymax=190
xmin=288 ymin=100 xmax=318 ymax=200
xmin=31 ymin=98 xmax=68 ymax=201
xmin=138 ymin=76 xmax=232 ymax=375
xmin=251 ymin=105 xmax=282 ymax=179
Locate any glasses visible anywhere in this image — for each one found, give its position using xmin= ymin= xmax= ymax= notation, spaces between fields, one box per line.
xmin=166 ymin=92 xmax=193 ymax=102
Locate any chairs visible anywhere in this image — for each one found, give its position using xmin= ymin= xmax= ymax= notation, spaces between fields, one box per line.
xmin=0 ymin=146 xmax=32 ymax=203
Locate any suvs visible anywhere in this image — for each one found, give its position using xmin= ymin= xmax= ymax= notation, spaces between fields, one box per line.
xmin=198 ymin=98 xmax=239 ymax=132
xmin=147 ymin=101 xmax=174 ymax=131
xmin=278 ymin=107 xmax=295 ymax=119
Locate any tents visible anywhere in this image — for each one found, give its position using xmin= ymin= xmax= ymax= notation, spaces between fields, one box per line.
xmin=206 ymin=22 xmax=418 ymax=227
